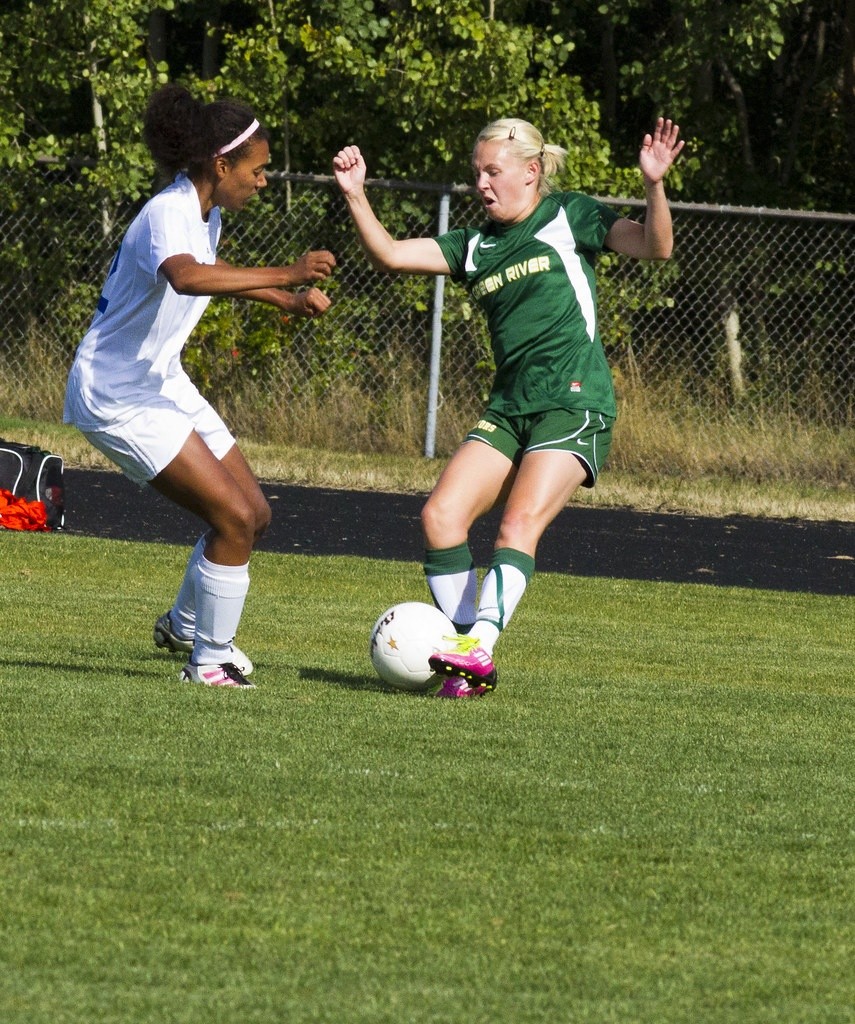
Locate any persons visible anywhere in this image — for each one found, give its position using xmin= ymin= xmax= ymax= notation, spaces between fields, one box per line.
xmin=334 ymin=118 xmax=684 ymax=696
xmin=62 ymin=87 xmax=336 ymax=690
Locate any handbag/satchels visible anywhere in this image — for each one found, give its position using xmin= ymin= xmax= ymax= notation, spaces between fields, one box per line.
xmin=0 ymin=438 xmax=65 ymax=530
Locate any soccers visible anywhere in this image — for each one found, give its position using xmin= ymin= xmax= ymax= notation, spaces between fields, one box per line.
xmin=369 ymin=602 xmax=458 ymax=691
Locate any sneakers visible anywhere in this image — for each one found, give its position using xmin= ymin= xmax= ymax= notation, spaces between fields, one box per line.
xmin=435 ymin=674 xmax=485 ymax=700
xmin=428 ymin=644 xmax=498 ymax=692
xmin=179 ymin=655 xmax=257 ymax=689
xmin=153 ymin=610 xmax=254 ymax=676
